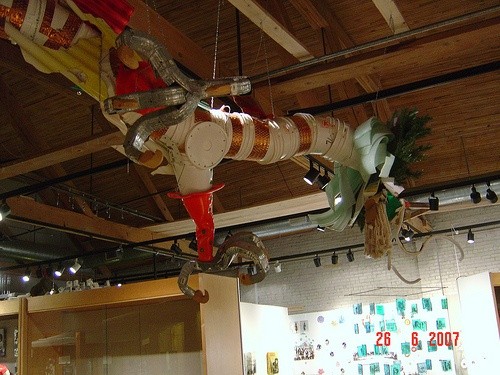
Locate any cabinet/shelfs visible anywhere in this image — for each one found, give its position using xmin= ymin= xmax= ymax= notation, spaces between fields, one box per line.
xmin=0 ymin=273 xmax=246 ymax=375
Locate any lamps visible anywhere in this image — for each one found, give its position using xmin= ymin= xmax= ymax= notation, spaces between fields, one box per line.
xmin=23 ymin=258 xmax=82 ymax=282
xmin=0 ymin=200 xmax=12 ymax=221
xmin=429 ymin=180 xmax=499 ymax=211
xmin=303 ymin=159 xmax=330 ymax=189
xmin=170 ymin=236 xmax=198 ymax=256
xmin=273 ymin=229 xmax=474 ymax=273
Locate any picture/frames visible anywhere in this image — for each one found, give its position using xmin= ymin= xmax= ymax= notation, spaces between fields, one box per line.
xmin=267 ymin=352 xmax=279 ymax=375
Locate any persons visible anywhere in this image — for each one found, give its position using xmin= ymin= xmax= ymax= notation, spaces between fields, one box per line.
xmin=273 ymin=359 xmax=278 ymax=369
xmin=298 ymin=347 xmax=313 ymax=360
